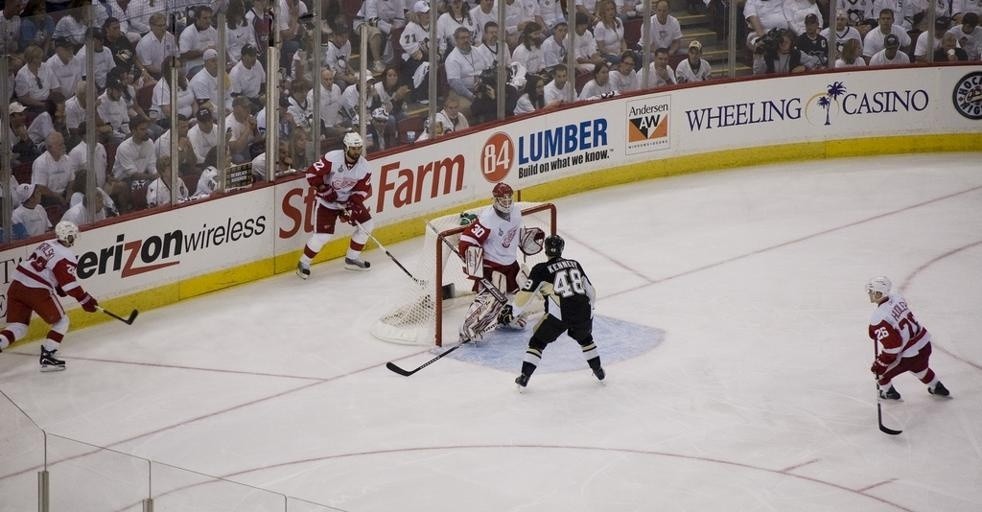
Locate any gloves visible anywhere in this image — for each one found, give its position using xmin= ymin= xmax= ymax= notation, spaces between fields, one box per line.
xmin=345 ymin=193 xmax=367 ymax=222
xmin=77 ymin=290 xmax=99 ymax=313
xmin=317 ymin=187 xmax=339 ymax=203
xmin=54 ymin=283 xmax=68 ymax=298
xmin=871 ymin=357 xmax=890 ymax=375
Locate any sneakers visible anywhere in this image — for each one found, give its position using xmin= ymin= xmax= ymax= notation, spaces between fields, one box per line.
xmin=296 ymin=260 xmax=312 ymax=276
xmin=344 ymin=257 xmax=371 ymax=269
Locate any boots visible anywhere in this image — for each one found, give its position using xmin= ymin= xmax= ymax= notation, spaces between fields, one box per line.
xmin=928 ymin=381 xmax=950 ymax=396
xmin=879 ymin=386 xmax=901 ymax=399
xmin=38 ymin=343 xmax=66 ymax=366
xmin=592 ymin=367 xmax=606 ymax=380
xmin=514 ymin=375 xmax=531 ymax=387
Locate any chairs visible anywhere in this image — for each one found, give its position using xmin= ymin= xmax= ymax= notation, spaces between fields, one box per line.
xmin=671 ymin=54 xmax=688 ymax=69
xmin=575 ymin=71 xmax=594 ymax=96
xmin=623 ymin=18 xmax=643 ymax=49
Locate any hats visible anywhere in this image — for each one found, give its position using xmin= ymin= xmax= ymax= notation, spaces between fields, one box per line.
xmin=688 ymin=40 xmax=703 ymax=50
xmin=15 ymin=183 xmax=36 ymax=203
xmin=54 ymin=35 xmax=77 ymax=49
xmin=803 ymin=13 xmax=819 ymax=24
xmin=85 ymin=26 xmax=111 ymax=41
xmin=934 ymin=17 xmax=947 ymax=30
xmin=885 ymin=34 xmax=899 ymax=51
xmin=413 ymin=1 xmax=432 ymax=14
xmin=104 ymin=76 xmax=129 ymax=92
xmin=9 ymin=100 xmax=28 ymax=115
xmin=195 ymin=43 xmax=377 ymax=122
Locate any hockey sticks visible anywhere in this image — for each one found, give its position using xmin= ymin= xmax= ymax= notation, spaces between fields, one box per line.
xmin=95 ymin=305 xmax=138 ymax=325
xmin=385 ymin=323 xmax=500 ymax=376
xmin=425 ymin=220 xmax=507 ymax=305
xmin=873 ymin=339 xmax=902 ymax=435
xmin=333 ymin=200 xmax=430 ymax=287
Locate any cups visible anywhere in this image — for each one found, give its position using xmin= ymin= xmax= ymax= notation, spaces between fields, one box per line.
xmin=946 ymin=49 xmax=956 ymax=62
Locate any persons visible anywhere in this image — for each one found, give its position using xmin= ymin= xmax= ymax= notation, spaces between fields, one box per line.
xmin=458 ymin=181 xmax=544 ymax=344
xmin=296 ymin=131 xmax=376 ymax=275
xmin=499 ymin=233 xmax=607 ymax=387
xmin=864 ymin=274 xmax=951 ymax=401
xmin=0 ymin=219 xmax=101 ymax=368
xmin=685 ymin=0 xmax=982 ymax=76
xmin=1 ymin=1 xmax=711 ymax=241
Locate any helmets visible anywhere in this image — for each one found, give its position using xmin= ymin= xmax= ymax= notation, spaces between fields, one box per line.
xmin=492 ymin=182 xmax=513 ymax=197
xmin=54 ymin=220 xmax=79 ymax=236
xmin=342 ymin=131 xmax=365 ymax=147
xmin=544 ymin=234 xmax=565 ymax=255
xmin=866 ymin=275 xmax=892 ymax=293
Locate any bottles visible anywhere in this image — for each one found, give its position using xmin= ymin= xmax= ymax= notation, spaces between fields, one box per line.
xmin=460 ymin=214 xmax=478 ymax=225
